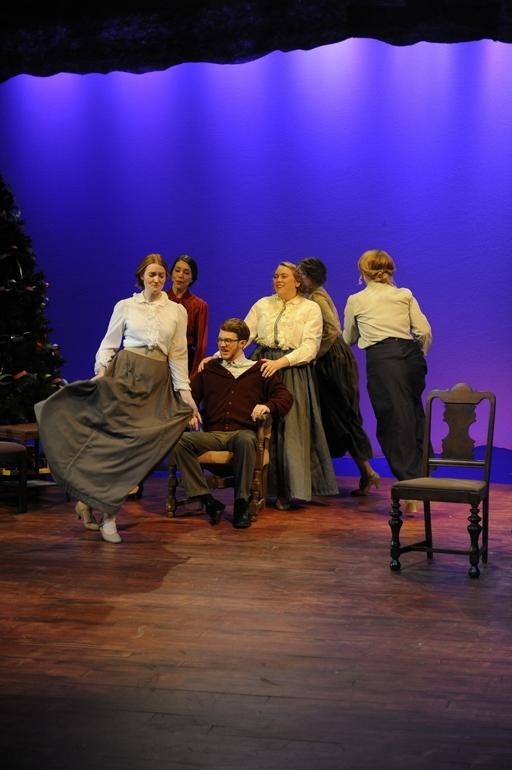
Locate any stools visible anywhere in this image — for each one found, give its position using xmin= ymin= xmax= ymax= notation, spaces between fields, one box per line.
xmin=0 ymin=442 xmax=27 ymax=512
xmin=0 ymin=423 xmax=39 ymax=472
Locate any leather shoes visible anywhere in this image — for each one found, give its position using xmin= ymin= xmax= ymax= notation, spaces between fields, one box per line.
xmin=99 ymin=518 xmax=123 ymax=544
xmin=272 ymin=496 xmax=292 ymax=511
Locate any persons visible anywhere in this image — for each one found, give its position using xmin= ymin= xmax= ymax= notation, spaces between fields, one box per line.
xmin=197 ymin=262 xmax=339 ymax=511
xmin=341 ymin=249 xmax=432 ymax=518
xmin=297 ymin=255 xmax=381 ymax=496
xmin=125 ymin=256 xmax=208 ymax=503
xmin=172 ymin=316 xmax=293 ymax=528
xmin=31 ymin=254 xmax=202 ymax=545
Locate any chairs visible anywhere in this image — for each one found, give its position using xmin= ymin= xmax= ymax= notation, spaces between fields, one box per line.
xmin=166 ymin=414 xmax=272 ymax=521
xmin=389 ymin=383 xmax=496 ymax=577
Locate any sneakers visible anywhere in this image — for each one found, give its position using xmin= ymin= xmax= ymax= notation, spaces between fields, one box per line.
xmin=232 ymin=500 xmax=252 ymax=528
xmin=404 ymin=503 xmax=418 ymax=514
xmin=208 ymin=499 xmax=227 ymax=525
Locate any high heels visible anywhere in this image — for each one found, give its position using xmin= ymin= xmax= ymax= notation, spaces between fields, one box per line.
xmin=350 ymin=472 xmax=381 ymax=497
xmin=73 ymin=501 xmax=99 ymax=532
